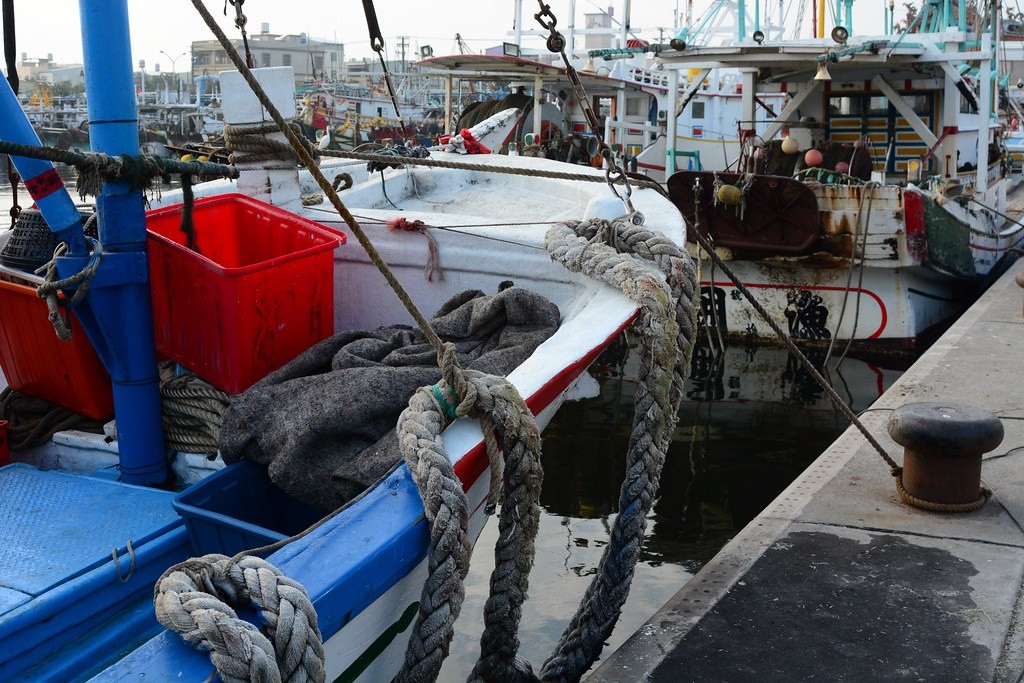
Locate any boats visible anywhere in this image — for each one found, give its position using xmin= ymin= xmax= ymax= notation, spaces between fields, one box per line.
xmin=0 ymin=0 xmax=1024 ymax=683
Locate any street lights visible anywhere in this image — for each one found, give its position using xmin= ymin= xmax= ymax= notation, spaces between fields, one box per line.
xmin=159 ymin=50 xmax=186 ymax=87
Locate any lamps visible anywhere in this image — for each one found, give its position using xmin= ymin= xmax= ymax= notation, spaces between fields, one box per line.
xmin=813 ymin=61 xmax=832 ymax=79
xmin=999 ymin=73 xmax=1011 ymax=89
xmin=503 ymin=42 xmax=521 ymax=57
xmin=752 ymin=30 xmax=765 ymax=45
xmin=581 ymin=50 xmax=597 ymax=74
xmin=670 ymin=28 xmax=690 ymax=51
xmin=832 ymin=25 xmax=852 ymax=46
xmin=959 ymin=59 xmax=971 ymax=76
xmin=419 ymin=45 xmax=433 ymax=58
xmin=1015 ymin=78 xmax=1024 ymax=89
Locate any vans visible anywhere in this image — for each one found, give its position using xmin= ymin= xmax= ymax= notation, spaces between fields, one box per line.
xmin=137 ymin=92 xmax=157 ymax=105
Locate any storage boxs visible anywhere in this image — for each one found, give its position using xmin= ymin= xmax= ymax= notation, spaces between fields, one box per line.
xmin=0 ymin=193 xmax=347 ymax=422
xmin=170 ymin=456 xmax=329 ymax=560
